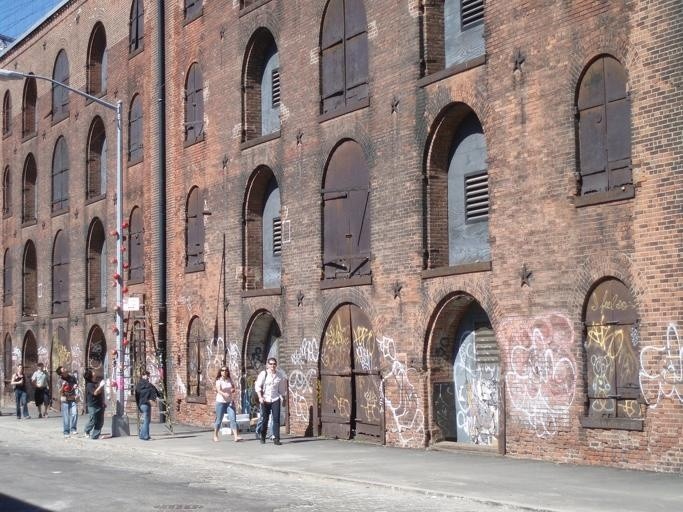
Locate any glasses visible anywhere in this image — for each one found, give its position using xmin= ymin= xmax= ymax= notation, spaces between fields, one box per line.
xmin=269 ymin=363 xmax=276 ymax=365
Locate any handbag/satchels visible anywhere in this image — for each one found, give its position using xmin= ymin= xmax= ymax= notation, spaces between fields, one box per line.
xmin=252 ymin=391 xmax=259 ymax=406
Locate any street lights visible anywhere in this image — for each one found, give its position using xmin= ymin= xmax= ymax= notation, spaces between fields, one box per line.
xmin=0 ymin=68 xmax=129 ymax=438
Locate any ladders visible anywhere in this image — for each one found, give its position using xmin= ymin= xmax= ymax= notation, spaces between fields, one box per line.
xmin=120 ymin=304 xmax=174 ymax=436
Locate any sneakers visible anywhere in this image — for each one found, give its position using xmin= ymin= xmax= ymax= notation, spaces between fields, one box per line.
xmin=261 ymin=432 xmax=265 ymax=444
xmin=274 ymin=439 xmax=281 ymax=445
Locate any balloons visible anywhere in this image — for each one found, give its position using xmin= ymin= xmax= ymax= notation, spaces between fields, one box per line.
xmin=106 ymin=220 xmax=130 ymax=388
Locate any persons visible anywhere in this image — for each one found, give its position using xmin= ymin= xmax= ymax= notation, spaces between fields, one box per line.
xmin=254 ymin=357 xmax=286 ymax=445
xmin=55 ymin=366 xmax=77 ymax=437
xmin=83 ymin=369 xmax=106 ymax=439
xmin=9 ymin=364 xmax=29 ymax=419
xmin=211 ymin=366 xmax=242 ymax=442
xmin=134 ymin=370 xmax=160 ymax=440
xmin=30 ymin=362 xmax=50 ymax=419
